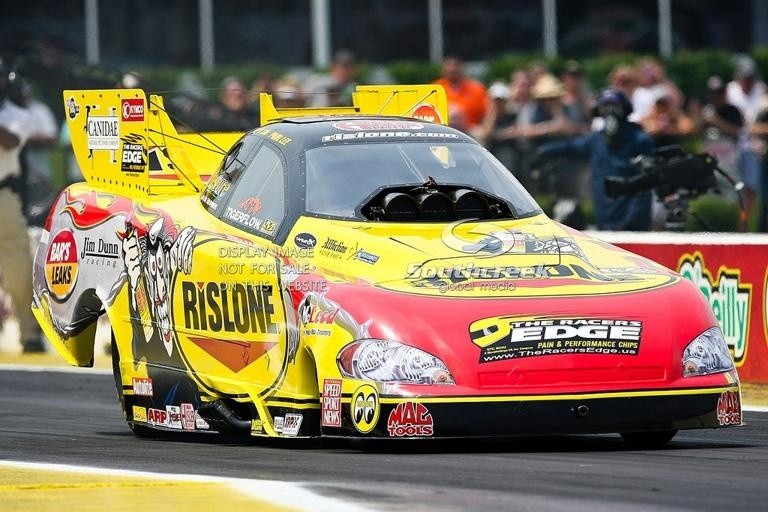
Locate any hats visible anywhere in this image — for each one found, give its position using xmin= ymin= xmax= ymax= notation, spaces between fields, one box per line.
xmin=599 ymin=89 xmax=625 ymax=109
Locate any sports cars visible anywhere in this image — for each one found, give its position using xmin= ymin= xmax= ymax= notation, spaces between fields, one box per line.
xmin=27 ymin=80 xmax=746 ymax=454
